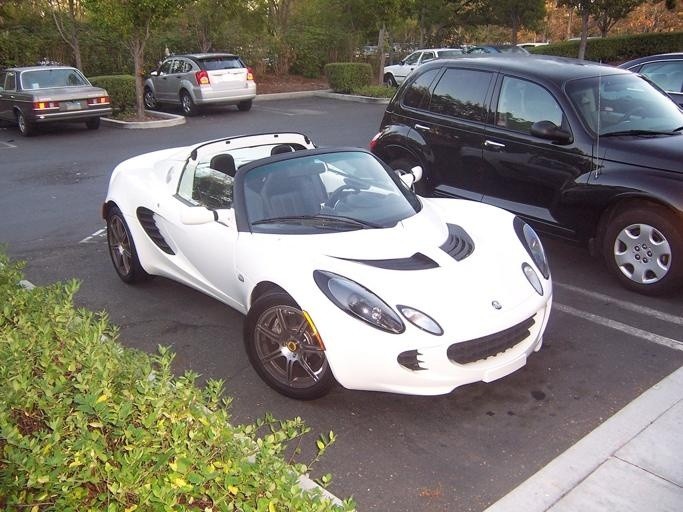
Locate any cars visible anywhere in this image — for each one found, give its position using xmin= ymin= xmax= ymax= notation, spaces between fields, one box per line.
xmin=143 ymin=53 xmax=257 ymax=117
xmin=0 ymin=66 xmax=112 ymax=137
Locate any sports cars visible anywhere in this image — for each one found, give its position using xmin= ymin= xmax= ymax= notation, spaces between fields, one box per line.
xmin=101 ymin=132 xmax=553 ymax=401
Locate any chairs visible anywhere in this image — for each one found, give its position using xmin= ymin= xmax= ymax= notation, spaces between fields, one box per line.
xmin=197 ymin=143 xmax=328 ymax=222
